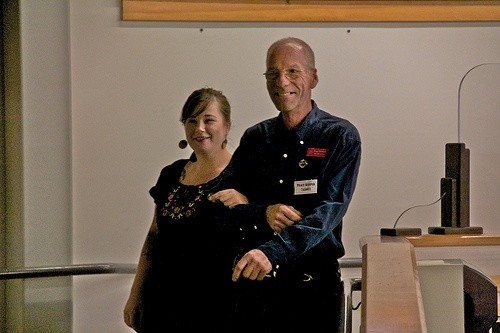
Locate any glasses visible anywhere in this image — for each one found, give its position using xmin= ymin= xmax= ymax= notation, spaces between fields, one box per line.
xmin=263 ymin=68 xmax=313 ymax=81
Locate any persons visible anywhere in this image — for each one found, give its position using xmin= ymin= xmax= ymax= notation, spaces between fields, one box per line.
xmin=204 ymin=36 xmax=362 ymax=333
xmin=123 ymin=87 xmax=250 ymax=333
